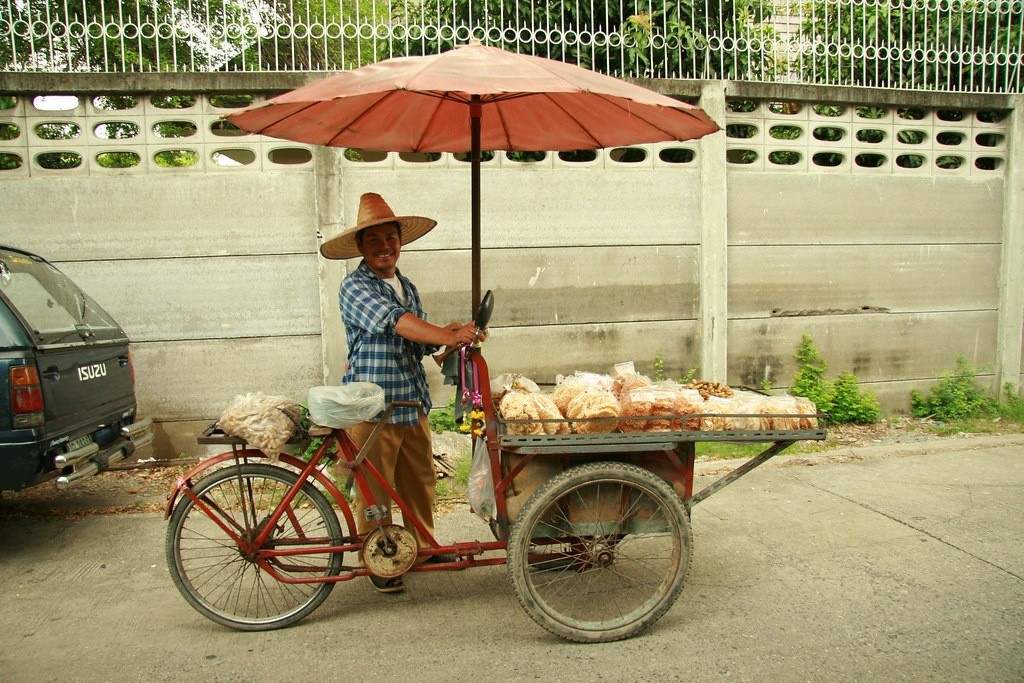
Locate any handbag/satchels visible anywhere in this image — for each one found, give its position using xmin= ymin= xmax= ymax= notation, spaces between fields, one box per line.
xmin=466 ymin=434 xmax=505 ymax=523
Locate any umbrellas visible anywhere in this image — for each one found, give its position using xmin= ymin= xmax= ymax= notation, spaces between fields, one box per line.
xmin=222 ymin=44 xmax=722 ymax=355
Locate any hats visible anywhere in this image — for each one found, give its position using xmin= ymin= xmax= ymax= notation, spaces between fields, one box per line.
xmin=316 ymin=193 xmax=437 ymax=259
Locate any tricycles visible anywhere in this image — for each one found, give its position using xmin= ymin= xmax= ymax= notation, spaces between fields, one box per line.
xmin=162 ymin=349 xmax=828 ymax=644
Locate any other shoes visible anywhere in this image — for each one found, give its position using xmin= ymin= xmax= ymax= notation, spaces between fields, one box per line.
xmin=424 ymin=554 xmax=463 ymax=565
xmin=364 ymin=567 xmax=408 ymax=594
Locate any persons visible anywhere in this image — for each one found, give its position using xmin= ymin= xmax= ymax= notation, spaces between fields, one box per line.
xmin=321 ymin=192 xmax=486 ymax=593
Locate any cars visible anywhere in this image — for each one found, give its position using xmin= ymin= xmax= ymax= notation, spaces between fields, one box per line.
xmin=0 ymin=243 xmax=141 ymax=499
